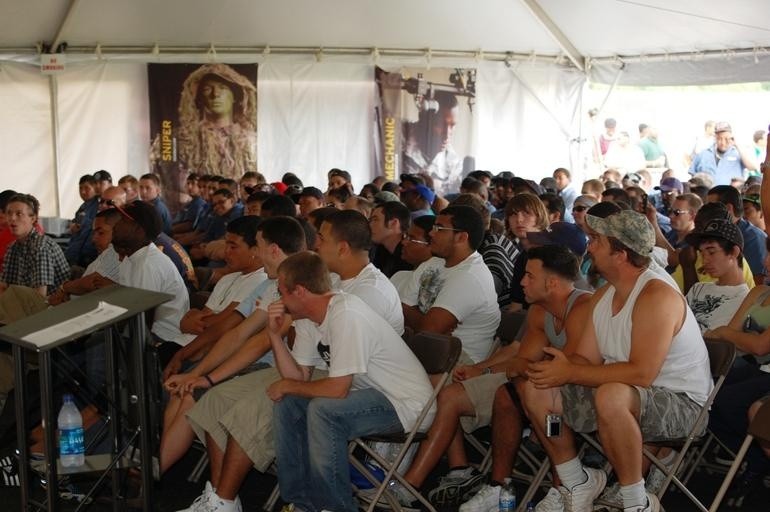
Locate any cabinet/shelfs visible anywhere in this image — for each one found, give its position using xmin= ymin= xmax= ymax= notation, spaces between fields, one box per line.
xmin=1 ymin=286 xmax=176 ymax=512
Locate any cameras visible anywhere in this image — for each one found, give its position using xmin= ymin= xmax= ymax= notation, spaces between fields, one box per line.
xmin=545 ymin=413 xmax=563 ymax=437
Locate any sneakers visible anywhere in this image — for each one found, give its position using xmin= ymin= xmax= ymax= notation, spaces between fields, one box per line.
xmin=460 ymin=476 xmax=516 ymax=511
xmin=356 ymin=481 xmax=422 ymax=512
xmin=428 ymin=465 xmax=486 ymax=507
xmin=116 ymin=439 xmax=159 ymax=480
xmin=531 ymin=450 xmax=684 ymax=511
xmin=176 ymin=480 xmax=243 ymax=511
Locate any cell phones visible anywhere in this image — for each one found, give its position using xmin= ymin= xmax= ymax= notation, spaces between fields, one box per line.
xmin=642 ymin=194 xmax=648 ymax=213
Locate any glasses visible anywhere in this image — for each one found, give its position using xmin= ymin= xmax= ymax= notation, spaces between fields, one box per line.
xmin=433 ymin=225 xmax=461 ymax=232
xmin=327 ymin=201 xmax=346 ymax=208
xmin=667 ymin=208 xmax=689 ymax=215
xmin=98 ymin=197 xmax=115 ymax=206
xmin=402 ymin=234 xmax=428 ymax=243
xmin=573 ymin=205 xmax=588 ymax=211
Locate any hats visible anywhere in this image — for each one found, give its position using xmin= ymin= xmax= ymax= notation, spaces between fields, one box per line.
xmin=685 ymin=218 xmax=743 ymax=249
xmin=491 ymin=170 xmax=540 ymax=195
xmin=89 ymin=170 xmax=112 ymax=181
xmin=524 ymin=221 xmax=584 ymax=255
xmin=654 ymin=177 xmax=683 ymax=195
xmin=716 ymin=123 xmax=730 ymax=132
xmin=245 ymin=183 xmax=275 ymax=196
xmin=292 ymin=186 xmax=323 ymax=204
xmin=585 ymin=211 xmax=656 ymax=256
xmin=400 ymin=173 xmax=426 ymax=185
xmin=407 ymin=186 xmax=434 ymax=205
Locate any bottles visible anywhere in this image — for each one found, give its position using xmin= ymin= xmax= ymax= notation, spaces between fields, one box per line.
xmin=499 ymin=478 xmax=536 ymax=511
xmin=58 ymin=394 xmax=84 ymax=467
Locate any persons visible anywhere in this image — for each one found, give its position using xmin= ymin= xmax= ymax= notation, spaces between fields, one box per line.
xmin=150 ymin=63 xmax=257 ymax=172
xmin=1 ymin=118 xmax=769 ymax=512
xmin=401 ymin=92 xmax=464 ymax=186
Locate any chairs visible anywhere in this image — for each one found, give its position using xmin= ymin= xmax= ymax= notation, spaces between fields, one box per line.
xmin=188 ymin=309 xmax=753 ymax=511
